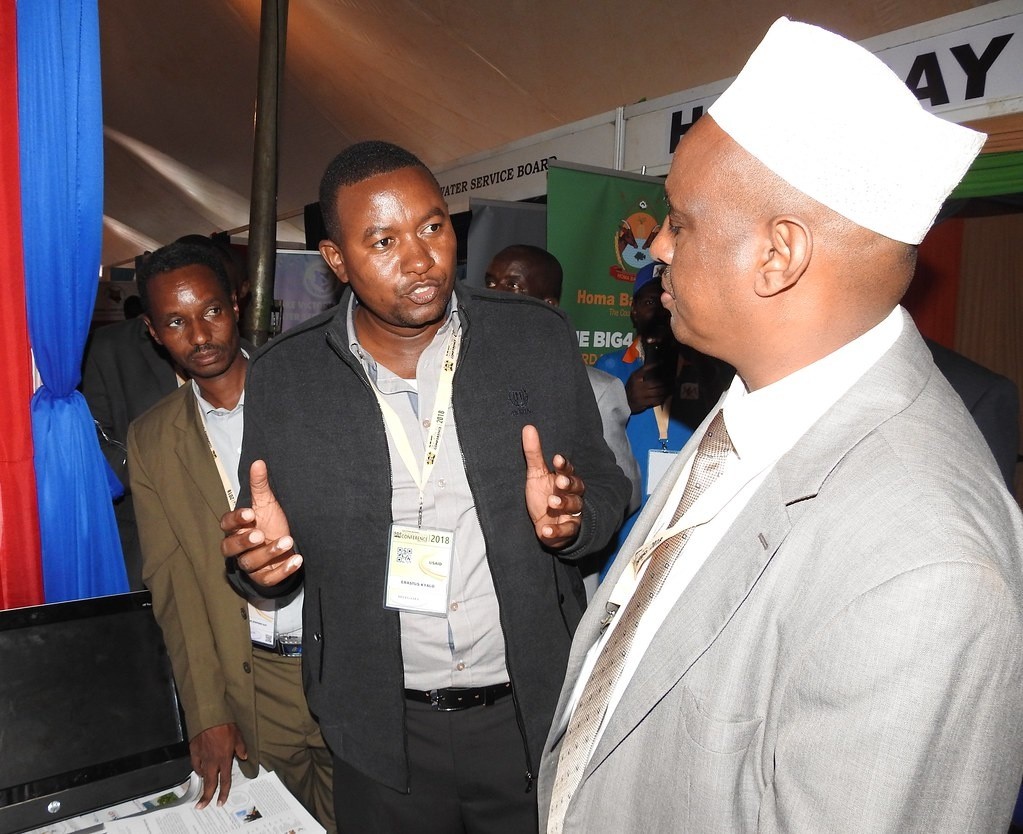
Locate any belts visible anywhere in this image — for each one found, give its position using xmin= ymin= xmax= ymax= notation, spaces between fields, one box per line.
xmin=252 ymin=636 xmax=303 ymax=658
xmin=404 ymin=682 xmax=510 ymax=712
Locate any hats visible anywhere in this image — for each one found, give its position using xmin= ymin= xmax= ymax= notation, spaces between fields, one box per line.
xmin=708 ymin=16 xmax=988 ymax=245
xmin=632 ymin=262 xmax=667 ymax=296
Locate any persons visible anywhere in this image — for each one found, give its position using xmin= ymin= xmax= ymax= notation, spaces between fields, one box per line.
xmin=80 ymin=227 xmax=742 ymax=834
xmin=222 ymin=139 xmax=633 ymax=834
xmin=535 ymin=17 xmax=1023 ymax=834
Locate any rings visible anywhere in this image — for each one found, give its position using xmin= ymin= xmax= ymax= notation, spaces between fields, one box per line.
xmin=572 ymin=511 xmax=582 ymax=517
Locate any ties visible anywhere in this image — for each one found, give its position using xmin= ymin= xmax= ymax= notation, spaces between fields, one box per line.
xmin=546 ymin=413 xmax=735 ymax=834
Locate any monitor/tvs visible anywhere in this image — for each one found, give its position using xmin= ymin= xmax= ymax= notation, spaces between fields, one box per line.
xmin=0 ymin=590 xmax=193 ymax=834
